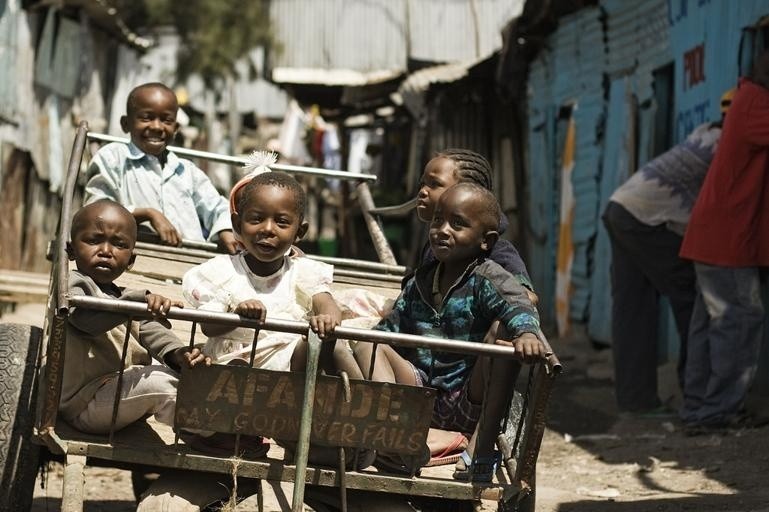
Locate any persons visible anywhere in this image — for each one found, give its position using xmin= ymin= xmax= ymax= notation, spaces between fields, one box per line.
xmin=57 ymin=197 xmax=271 ymax=458
xmin=677 ymin=54 xmax=767 ymax=435
xmin=181 ymin=171 xmax=376 ymax=470
xmin=354 ymin=180 xmax=547 ymax=469
xmin=598 ymin=89 xmax=698 ymax=421
xmin=228 ymin=149 xmax=279 ymax=248
xmin=83 ymin=83 xmax=241 ymax=254
xmin=400 ymin=146 xmax=540 ymax=311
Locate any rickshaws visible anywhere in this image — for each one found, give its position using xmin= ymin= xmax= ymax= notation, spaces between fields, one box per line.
xmin=2 ymin=120 xmax=564 ymax=512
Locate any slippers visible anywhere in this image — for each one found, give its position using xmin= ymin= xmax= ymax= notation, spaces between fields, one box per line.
xmin=426 ymin=428 xmax=494 ymax=482
